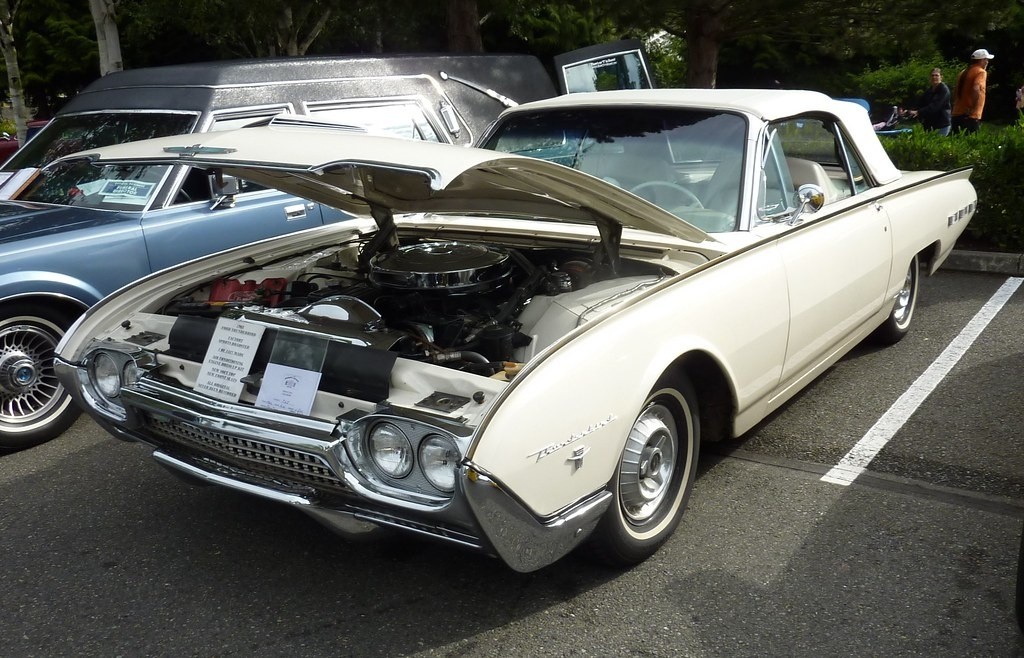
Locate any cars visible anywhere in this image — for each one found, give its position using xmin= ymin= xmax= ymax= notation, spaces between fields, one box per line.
xmin=55 ymin=88 xmax=979 ymax=573
xmin=0 ymin=120 xmax=51 ymax=164
xmin=0 ymin=37 xmax=657 ymax=449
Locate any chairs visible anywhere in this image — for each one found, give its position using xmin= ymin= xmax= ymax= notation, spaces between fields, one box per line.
xmin=702 ymin=154 xmax=841 ymax=215
xmin=577 ymin=152 xmax=672 ymax=203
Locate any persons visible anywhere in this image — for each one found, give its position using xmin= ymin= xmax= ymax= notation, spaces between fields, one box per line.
xmin=952 ymin=49 xmax=995 ymax=134
xmin=900 ymin=67 xmax=951 ymax=136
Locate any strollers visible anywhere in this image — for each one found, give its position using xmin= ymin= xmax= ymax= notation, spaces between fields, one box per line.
xmin=872 ymin=106 xmax=913 ymax=132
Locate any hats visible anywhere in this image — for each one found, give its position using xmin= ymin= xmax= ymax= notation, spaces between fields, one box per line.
xmin=971 ymin=48 xmax=995 ymax=60
